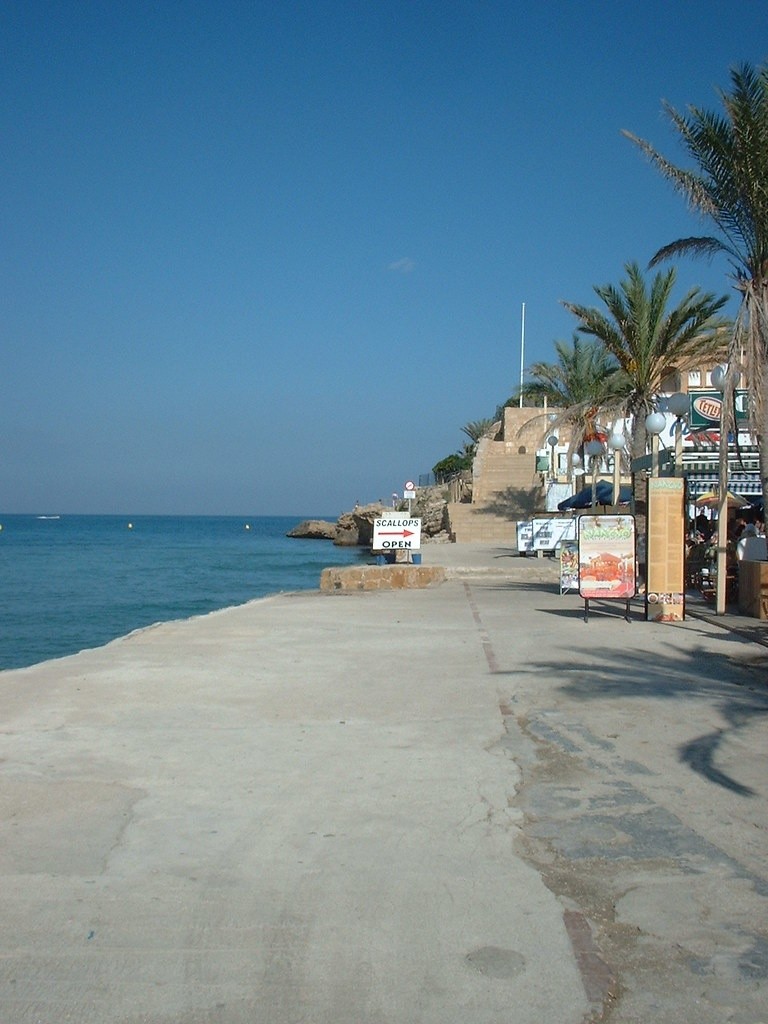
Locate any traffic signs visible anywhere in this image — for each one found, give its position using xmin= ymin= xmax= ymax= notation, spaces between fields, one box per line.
xmin=373 ymin=518 xmax=422 ymax=551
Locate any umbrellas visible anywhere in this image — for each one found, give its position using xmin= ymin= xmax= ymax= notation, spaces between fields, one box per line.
xmin=691 ymin=486 xmax=754 ymax=512
xmin=684 ymin=430 xmax=737 ymax=443
xmin=558 ymin=480 xmax=634 ymax=511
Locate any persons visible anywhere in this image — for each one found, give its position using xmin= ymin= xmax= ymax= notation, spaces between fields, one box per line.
xmin=632 ymin=506 xmax=768 ymax=596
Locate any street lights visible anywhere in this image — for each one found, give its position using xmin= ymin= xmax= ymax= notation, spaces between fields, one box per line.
xmin=572 ymin=453 xmax=580 ymax=496
xmin=589 ymin=441 xmax=601 ymax=508
xmin=668 ymin=393 xmax=690 ymax=477
xmin=548 ymin=436 xmax=558 ymax=475
xmin=645 ymin=412 xmax=666 ymax=477
xmin=711 ymin=364 xmax=741 ymax=617
xmin=609 ymin=435 xmax=623 ymax=507
